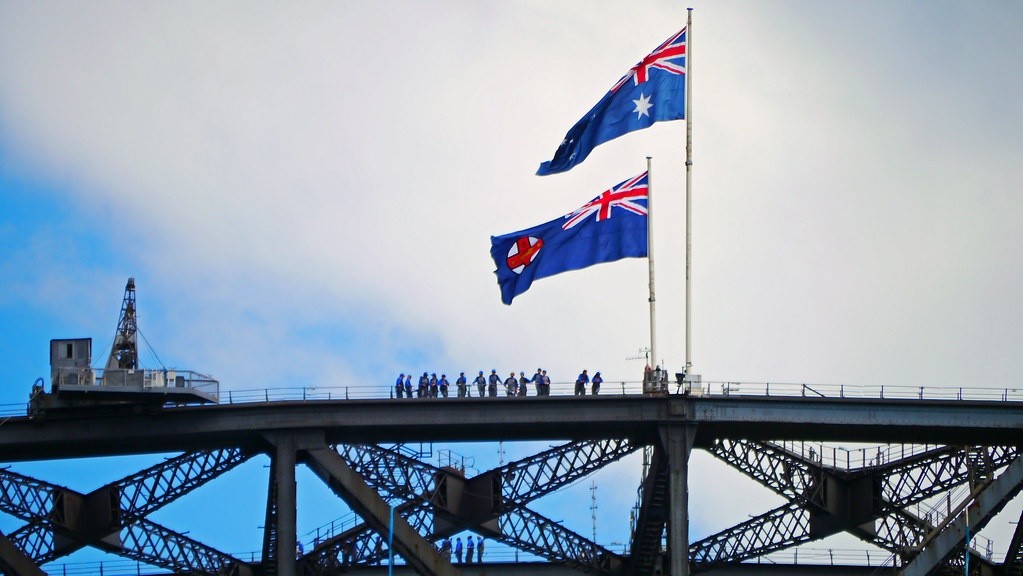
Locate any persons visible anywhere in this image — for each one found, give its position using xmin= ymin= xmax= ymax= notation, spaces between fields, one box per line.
xmin=530 ymin=368 xmax=542 ymax=396
xmin=429 ymin=373 xmax=438 ymax=398
xmin=396 ymin=374 xmax=406 ymax=398
xmin=539 ymin=370 xmax=550 ymax=396
xmin=655 ymin=366 xmax=661 ymax=379
xmin=575 ymin=370 xmax=589 ymax=395
xmin=503 ymin=372 xmax=518 ymax=397
xmin=296 ymin=535 xmax=381 ymax=566
xmin=592 ymin=372 xmax=603 ymax=396
xmin=405 ymin=375 xmax=413 ymax=398
xmin=488 ymin=369 xmax=502 ymax=397
xmin=417 ymin=372 xmax=429 ymax=398
xmin=429 ymin=536 xmax=485 ymax=564
xmin=518 ymin=372 xmax=530 ymax=396
xmin=644 ymin=365 xmax=651 ymax=381
xmin=436 ymin=374 xmax=449 ymax=398
xmin=456 ymin=372 xmax=466 ymax=398
xmin=472 ymin=371 xmax=486 ymax=397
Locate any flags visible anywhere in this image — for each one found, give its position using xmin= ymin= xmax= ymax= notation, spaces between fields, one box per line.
xmin=536 ymin=25 xmax=687 ymax=176
xmin=490 ymin=171 xmax=648 ymax=306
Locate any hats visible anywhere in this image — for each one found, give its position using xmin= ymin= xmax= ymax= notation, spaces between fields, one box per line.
xmin=424 ymin=372 xmax=428 ymax=376
xmin=460 ymin=372 xmax=464 ymax=376
xmin=442 ymin=375 xmax=446 ymax=377
xmin=597 ymin=372 xmax=601 ymax=375
xmin=479 ymin=371 xmax=483 ymax=375
xmin=400 ymin=374 xmax=405 ymax=377
xmin=431 ymin=373 xmax=436 ymax=376
xmin=538 ymin=368 xmax=541 ymax=371
xmin=492 ymin=370 xmax=496 ymax=372
